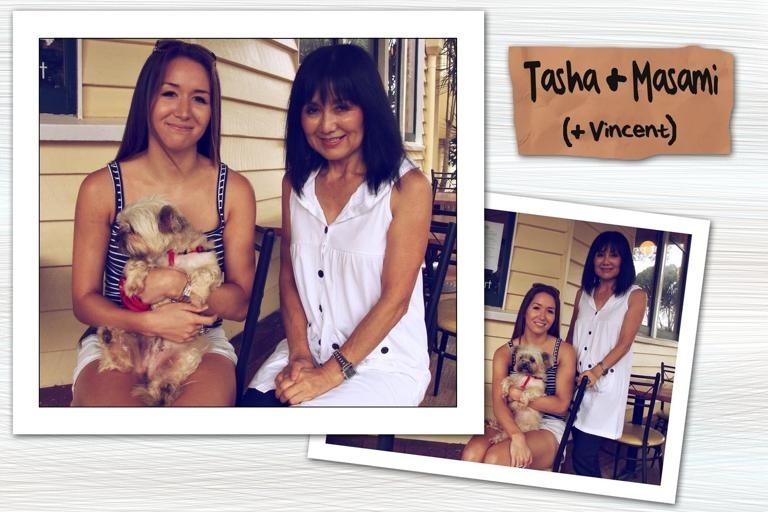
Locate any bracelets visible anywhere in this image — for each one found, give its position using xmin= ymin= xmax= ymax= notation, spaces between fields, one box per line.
xmin=596 ymin=361 xmax=608 ymax=377
xmin=178 ymin=280 xmax=194 ymax=306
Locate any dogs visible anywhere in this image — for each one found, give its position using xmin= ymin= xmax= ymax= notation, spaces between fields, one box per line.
xmin=486 ymin=344 xmax=554 ymax=445
xmin=97 ymin=196 xmax=225 ymax=407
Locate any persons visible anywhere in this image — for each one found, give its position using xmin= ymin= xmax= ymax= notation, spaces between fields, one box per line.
xmin=67 ymin=38 xmax=257 ymax=409
xmin=459 ymin=283 xmax=578 ymax=470
xmin=563 ymin=229 xmax=648 ymax=480
xmin=239 ymin=44 xmax=434 ymax=407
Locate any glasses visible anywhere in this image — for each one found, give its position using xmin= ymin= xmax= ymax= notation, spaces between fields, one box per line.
xmin=151 ymin=39 xmax=216 ymax=61
xmin=532 ymin=283 xmax=560 ymax=296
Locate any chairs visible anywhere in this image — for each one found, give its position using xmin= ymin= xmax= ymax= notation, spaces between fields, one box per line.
xmin=220 ymin=170 xmax=675 ymax=484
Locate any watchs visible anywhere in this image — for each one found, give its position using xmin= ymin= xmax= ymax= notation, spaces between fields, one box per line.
xmin=331 ymin=351 xmax=357 ymax=381
